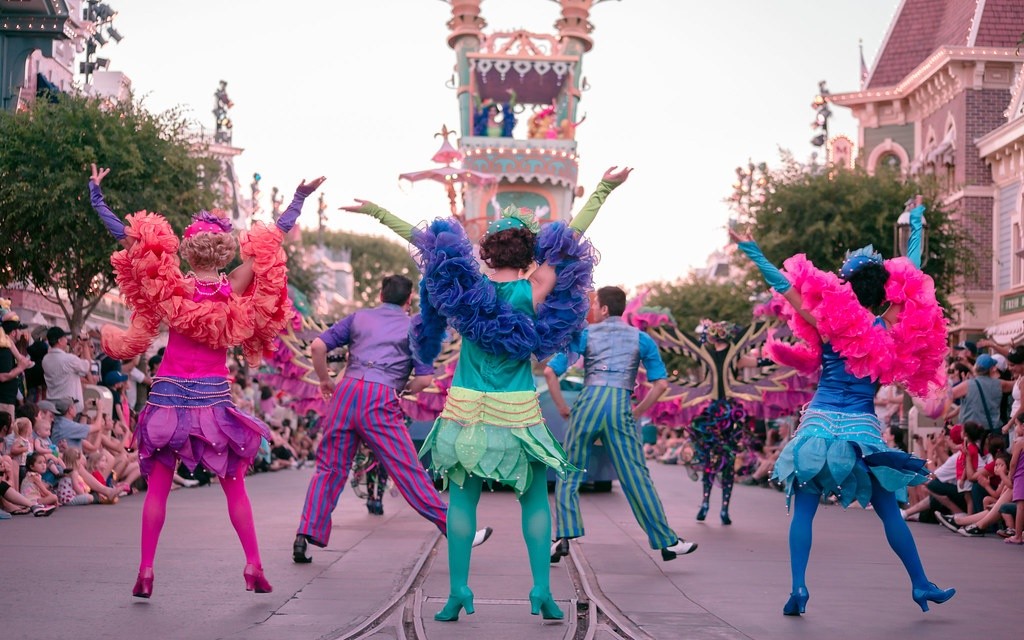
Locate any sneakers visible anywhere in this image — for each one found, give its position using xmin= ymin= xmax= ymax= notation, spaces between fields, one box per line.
xmin=935 ymin=511 xmax=986 ymax=537
xmin=31 ymin=505 xmax=56 ymax=517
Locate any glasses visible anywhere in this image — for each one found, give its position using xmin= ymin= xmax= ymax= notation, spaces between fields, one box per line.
xmin=1006 ymin=347 xmax=1021 ymax=357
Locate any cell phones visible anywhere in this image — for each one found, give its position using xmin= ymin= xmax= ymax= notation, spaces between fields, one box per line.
xmin=91 ymin=400 xmax=96 ymax=406
xmin=964 ymin=437 xmax=968 ymax=447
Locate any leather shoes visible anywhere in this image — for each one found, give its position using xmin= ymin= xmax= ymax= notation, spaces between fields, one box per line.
xmin=472 ymin=527 xmax=493 ymax=547
xmin=662 ymin=540 xmax=697 ymax=561
xmin=551 ymin=538 xmax=568 ymax=563
xmin=292 ymin=538 xmax=313 ymax=564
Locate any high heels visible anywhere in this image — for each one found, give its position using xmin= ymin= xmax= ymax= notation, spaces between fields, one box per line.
xmin=911 ymin=585 xmax=955 ymax=613
xmin=243 ymin=563 xmax=273 ymax=594
xmin=528 ymin=589 xmax=565 ymax=621
xmin=721 ymin=510 xmax=732 ymax=524
xmin=133 ymin=567 xmax=154 ymax=599
xmin=697 ymin=503 xmax=708 ymax=520
xmin=782 ymin=586 xmax=809 ymax=616
xmin=365 ymin=500 xmax=385 ymax=514
xmin=434 ymin=586 xmax=474 ymax=621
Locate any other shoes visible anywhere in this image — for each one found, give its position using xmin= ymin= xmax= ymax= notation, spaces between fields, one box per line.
xmin=645 ymin=454 xmax=771 ymax=488
xmin=185 ymin=479 xmax=200 ymax=488
xmin=295 ymin=456 xmax=306 ymax=470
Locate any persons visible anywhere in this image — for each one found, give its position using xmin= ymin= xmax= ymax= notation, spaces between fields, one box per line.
xmin=88 ymin=164 xmax=328 ymax=598
xmin=641 ymin=366 xmax=908 ymax=511
xmin=337 ymin=166 xmax=636 ymax=622
xmin=900 ymin=325 xmax=1024 ymax=545
xmin=146 ymin=336 xmax=395 ymax=516
xmin=729 ymin=194 xmax=956 ymax=615
xmin=292 ymin=275 xmax=493 ymax=564
xmin=474 ymin=88 xmax=586 ymax=140
xmin=693 ymin=318 xmax=745 ymax=524
xmin=551 ymin=286 xmax=698 ymax=564
xmin=0 ymin=297 xmax=153 ymax=520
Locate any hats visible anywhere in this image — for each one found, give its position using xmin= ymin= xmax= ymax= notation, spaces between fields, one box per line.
xmin=953 ymin=341 xmax=1008 ymax=372
xmin=105 ymin=371 xmax=128 ymax=385
xmin=485 ymin=204 xmax=541 ymax=235
xmin=37 ymin=396 xmax=79 ymax=415
xmin=839 ymin=251 xmax=882 ymax=277
xmin=182 ymin=210 xmax=230 ymax=237
xmin=1 ymin=320 xmax=73 ymax=339
